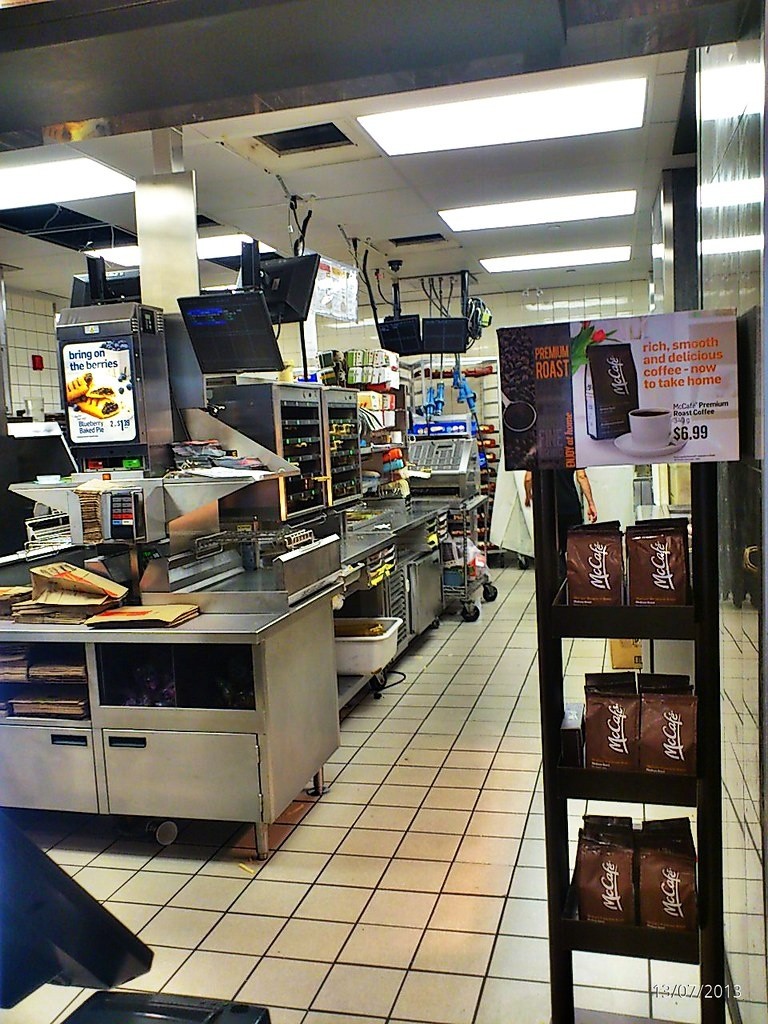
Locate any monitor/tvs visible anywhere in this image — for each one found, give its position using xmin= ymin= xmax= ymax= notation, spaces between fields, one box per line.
xmin=237 ymin=254 xmax=321 ymax=324
xmin=176 ymin=291 xmax=286 ymax=374
xmin=378 ymin=314 xmax=468 ymax=357
xmin=0 ymin=811 xmax=154 ymax=1012
xmin=69 ymin=269 xmax=141 ymax=308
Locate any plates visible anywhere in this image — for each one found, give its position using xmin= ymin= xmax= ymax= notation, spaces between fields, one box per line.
xmin=614 ymin=433 xmax=688 ymax=458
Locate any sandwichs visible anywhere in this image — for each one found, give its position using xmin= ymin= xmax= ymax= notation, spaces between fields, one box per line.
xmin=67 ymin=373 xmax=92 ymax=401
xmin=77 ymin=398 xmax=119 ymax=418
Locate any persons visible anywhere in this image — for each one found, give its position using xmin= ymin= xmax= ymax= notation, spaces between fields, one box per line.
xmin=524 ymin=467 xmax=598 ymax=553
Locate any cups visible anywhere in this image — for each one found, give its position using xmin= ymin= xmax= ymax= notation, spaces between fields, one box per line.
xmin=25 ymin=397 xmax=45 ymax=423
xmin=629 ymin=406 xmax=671 ymax=449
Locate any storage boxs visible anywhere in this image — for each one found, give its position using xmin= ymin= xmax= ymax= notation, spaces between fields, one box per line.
xmin=320 ymin=347 xmax=400 ymax=430
xmin=576 ymin=815 xmax=698 ymax=932
xmin=333 ymin=618 xmax=403 ymax=675
xmin=567 ymin=516 xmax=692 ymax=607
xmin=559 ymin=671 xmax=699 ymax=778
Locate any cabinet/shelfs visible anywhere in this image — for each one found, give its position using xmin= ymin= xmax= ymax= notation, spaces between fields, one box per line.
xmin=214 ymin=381 xmax=327 ymax=523
xmin=410 ymin=495 xmax=498 ymax=623
xmin=323 ymin=386 xmax=363 ymax=506
xmin=498 ymin=306 xmax=744 ymax=1024
xmin=415 ymin=355 xmax=508 ymax=568
